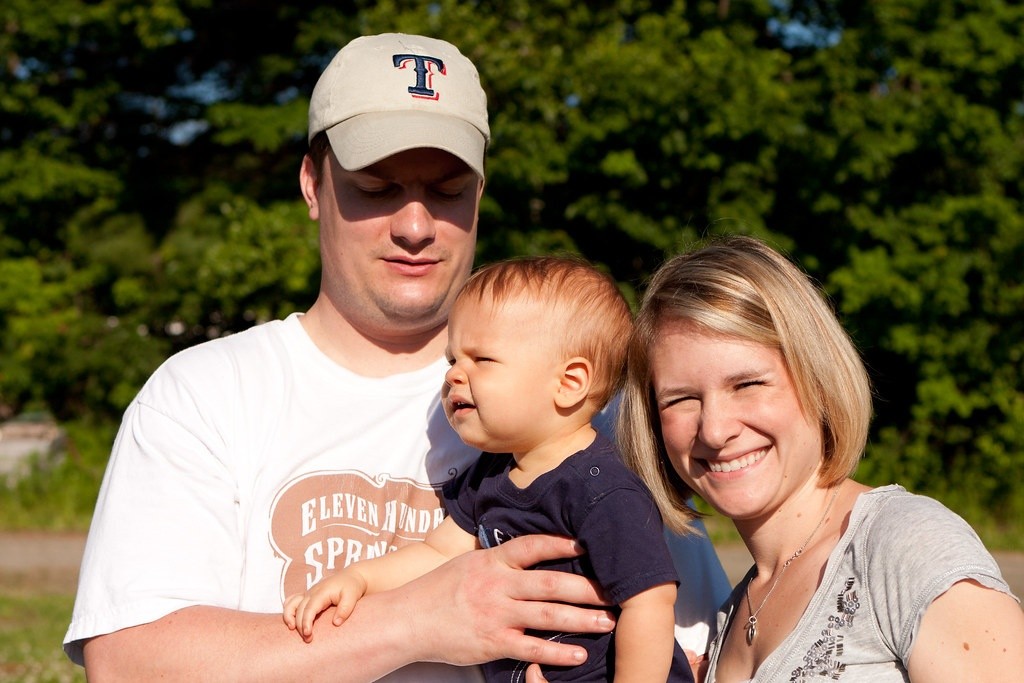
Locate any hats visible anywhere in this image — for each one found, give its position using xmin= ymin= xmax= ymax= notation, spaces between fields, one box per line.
xmin=310 ymin=33 xmax=491 ymax=181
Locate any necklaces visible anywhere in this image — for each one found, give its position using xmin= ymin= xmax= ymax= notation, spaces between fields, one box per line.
xmin=745 ymin=496 xmax=834 ymax=645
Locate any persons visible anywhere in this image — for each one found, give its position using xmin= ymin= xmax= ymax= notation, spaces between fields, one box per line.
xmin=284 ymin=258 xmax=694 ymax=683
xmin=617 ymin=235 xmax=1024 ymax=683
xmin=63 ymin=33 xmax=733 ymax=683
